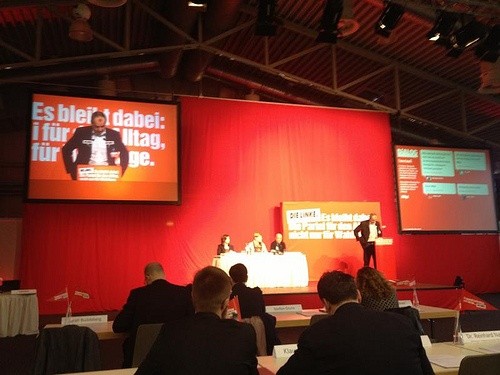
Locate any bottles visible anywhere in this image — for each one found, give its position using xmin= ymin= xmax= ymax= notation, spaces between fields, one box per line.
xmin=413 ymin=287 xmax=419 ymax=306
xmin=453 ymin=311 xmax=463 ymax=344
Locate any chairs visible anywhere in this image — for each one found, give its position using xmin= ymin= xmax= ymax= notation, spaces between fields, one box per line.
xmin=25 ymin=324 xmax=103 ymax=375
xmin=213 ymin=256 xmax=221 ymax=268
xmin=457 ymin=352 xmax=500 ymax=375
xmin=131 ymin=323 xmax=163 ymax=368
xmin=387 ymin=306 xmax=424 ymax=335
xmin=239 ymin=316 xmax=267 ymax=357
xmin=238 ymin=285 xmax=280 ymax=357
xmin=459 ymin=308 xmax=500 ymax=332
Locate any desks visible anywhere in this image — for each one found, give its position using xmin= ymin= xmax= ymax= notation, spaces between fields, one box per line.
xmin=219 ymin=251 xmax=309 ymax=288
xmin=0 ymin=292 xmax=41 ymax=337
xmin=43 ymin=303 xmax=500 ymax=375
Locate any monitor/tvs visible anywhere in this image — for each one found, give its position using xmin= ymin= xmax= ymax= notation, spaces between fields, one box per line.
xmin=23 ymin=90 xmax=182 ymax=205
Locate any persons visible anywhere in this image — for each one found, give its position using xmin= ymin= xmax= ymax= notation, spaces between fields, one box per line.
xmin=271 ymin=233 xmax=286 ymax=252
xmin=133 ymin=265 xmax=260 ymax=375
xmin=354 ymin=214 xmax=382 ymax=270
xmin=245 ymin=233 xmax=268 ymax=253
xmin=357 ymin=265 xmax=399 ymax=311
xmin=216 ymin=234 xmax=234 ymax=255
xmin=229 ymin=264 xmax=265 ymax=319
xmin=276 ymin=270 xmax=436 ymax=375
xmin=112 ymin=261 xmax=195 ymax=369
xmin=62 ymin=111 xmax=129 ymax=181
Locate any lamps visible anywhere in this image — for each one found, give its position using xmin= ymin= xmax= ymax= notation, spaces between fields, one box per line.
xmin=471 ymin=24 xmax=500 ymax=63
xmin=254 ymin=0 xmax=285 ymax=36
xmin=315 ymin=0 xmax=344 ymax=46
xmin=425 ymin=9 xmax=460 ymax=48
xmin=451 ymin=19 xmax=492 ymax=49
xmin=373 ymin=0 xmax=404 ymax=39
xmin=67 ymin=2 xmax=94 ymax=42
